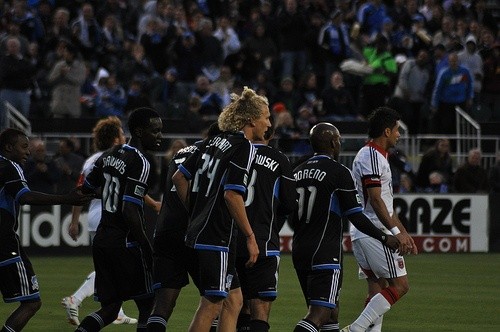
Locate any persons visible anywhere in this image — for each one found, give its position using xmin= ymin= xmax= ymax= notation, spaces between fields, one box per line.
xmin=237 ymin=122 xmax=301 ymax=332
xmin=0 ymin=128 xmax=96 ymax=332
xmin=173 ymin=90 xmax=272 ymax=332
xmin=340 ymin=107 xmax=418 ymax=332
xmin=0 ymin=0 xmax=499 ymax=197
xmin=61 ymin=107 xmax=163 ymax=332
xmin=290 ymin=122 xmax=400 ymax=332
xmin=147 ymin=122 xmax=223 ymax=332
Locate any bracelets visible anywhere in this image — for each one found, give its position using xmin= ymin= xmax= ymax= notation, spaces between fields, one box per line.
xmin=390 ymin=226 xmax=400 ymax=235
xmin=246 ymin=232 xmax=255 ymax=238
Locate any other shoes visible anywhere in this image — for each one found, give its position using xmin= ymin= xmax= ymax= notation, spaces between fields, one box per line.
xmin=339 ymin=325 xmax=351 ymax=332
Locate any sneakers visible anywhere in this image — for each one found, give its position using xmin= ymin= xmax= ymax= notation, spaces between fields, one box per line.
xmin=112 ymin=316 xmax=138 ymax=324
xmin=61 ymin=295 xmax=81 ymax=326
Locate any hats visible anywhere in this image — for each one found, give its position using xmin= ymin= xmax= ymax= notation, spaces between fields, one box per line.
xmin=395 ymin=55 xmax=407 ymax=63
xmin=183 ymin=32 xmax=192 ymax=40
xmin=413 ymin=15 xmax=423 ymax=21
xmin=282 ymin=76 xmax=294 ymax=84
xmin=272 ymin=104 xmax=285 ymax=113
xmin=330 ymin=8 xmax=341 ymax=21
xmin=168 ymin=68 xmax=177 ymax=74
